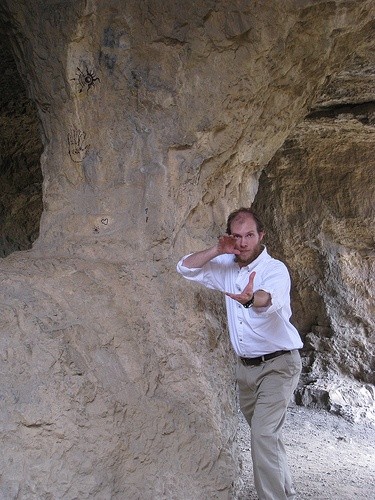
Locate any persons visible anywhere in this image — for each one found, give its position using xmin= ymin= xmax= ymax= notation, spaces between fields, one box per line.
xmin=176 ymin=207 xmax=305 ymax=500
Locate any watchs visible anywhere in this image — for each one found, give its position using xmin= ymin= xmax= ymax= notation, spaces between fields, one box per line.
xmin=242 ymin=293 xmax=255 ymax=309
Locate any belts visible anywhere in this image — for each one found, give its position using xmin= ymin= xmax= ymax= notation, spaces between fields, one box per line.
xmin=240 ymin=350 xmax=290 ymax=367
